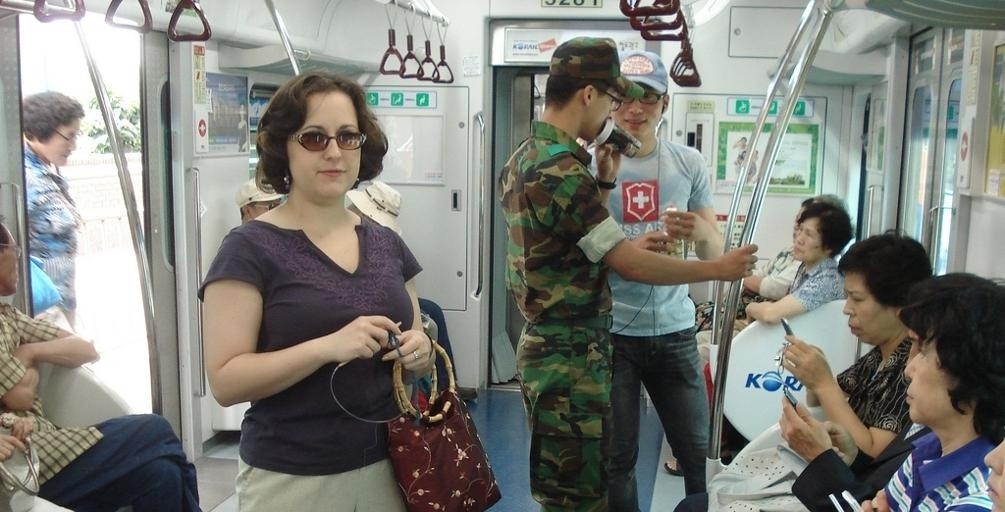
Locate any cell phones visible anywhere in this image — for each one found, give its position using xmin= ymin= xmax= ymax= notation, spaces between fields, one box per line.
xmin=784 ymin=388 xmax=800 ymax=408
xmin=781 ymin=318 xmax=795 ymax=335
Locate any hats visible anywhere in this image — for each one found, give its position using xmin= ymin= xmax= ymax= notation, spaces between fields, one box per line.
xmin=345 ymin=180 xmax=404 ymax=236
xmin=547 ymin=35 xmax=645 ymax=100
xmin=618 ymin=49 xmax=670 ymax=96
xmin=235 ymin=175 xmax=284 ymax=209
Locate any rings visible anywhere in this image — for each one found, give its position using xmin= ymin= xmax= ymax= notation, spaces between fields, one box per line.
xmin=413 ymin=351 xmax=419 ymax=360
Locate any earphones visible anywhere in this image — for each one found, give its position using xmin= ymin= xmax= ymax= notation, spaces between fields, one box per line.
xmin=661 ymin=104 xmax=666 ymax=113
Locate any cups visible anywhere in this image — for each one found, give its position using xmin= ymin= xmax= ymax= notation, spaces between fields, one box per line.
xmin=593 ymin=116 xmax=642 ymax=158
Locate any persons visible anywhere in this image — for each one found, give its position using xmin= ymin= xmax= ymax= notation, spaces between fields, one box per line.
xmin=497 ymin=37 xmax=759 ymax=510
xmin=0 ymin=415 xmax=78 ymax=511
xmin=580 ymin=49 xmax=723 ymax=510
xmin=23 ymin=89 xmax=86 ymax=336
xmin=0 ymin=212 xmax=204 ymax=511
xmin=732 ymin=135 xmax=759 ymax=185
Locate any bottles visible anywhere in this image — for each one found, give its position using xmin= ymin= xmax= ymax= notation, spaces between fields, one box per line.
xmin=661 ymin=207 xmax=684 ymax=260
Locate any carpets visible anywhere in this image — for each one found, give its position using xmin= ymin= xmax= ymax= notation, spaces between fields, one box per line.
xmin=192 ymin=454 xmax=240 ymax=512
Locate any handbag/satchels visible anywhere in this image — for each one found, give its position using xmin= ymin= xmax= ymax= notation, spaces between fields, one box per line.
xmin=0 ymin=433 xmax=41 ymax=512
xmin=381 ymin=339 xmax=507 ymax=512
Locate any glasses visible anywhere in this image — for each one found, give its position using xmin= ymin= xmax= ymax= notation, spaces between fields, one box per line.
xmin=0 ymin=243 xmax=23 ymax=259
xmin=587 ymin=81 xmax=623 ymax=112
xmin=50 ymin=125 xmax=86 ymax=146
xmin=281 ymin=128 xmax=367 ymax=153
xmin=622 ymin=92 xmax=666 ymax=106
